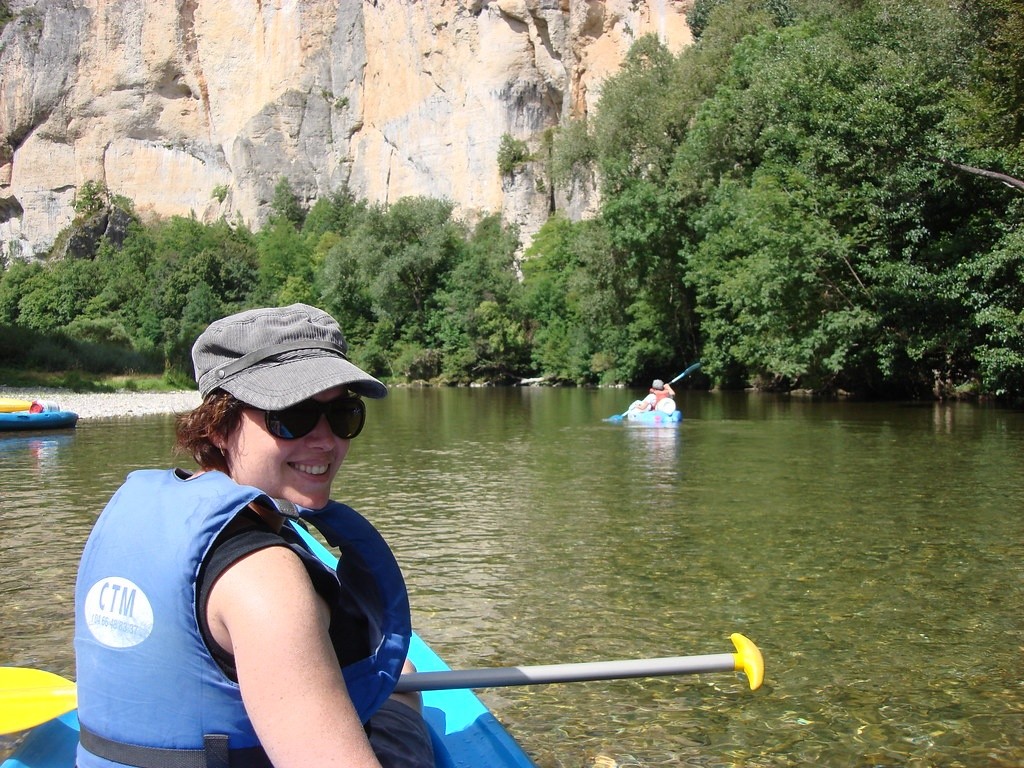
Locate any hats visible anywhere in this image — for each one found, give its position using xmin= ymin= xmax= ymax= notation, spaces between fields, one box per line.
xmin=652 ymin=379 xmax=664 ymax=390
xmin=192 ymin=303 xmax=388 ymax=410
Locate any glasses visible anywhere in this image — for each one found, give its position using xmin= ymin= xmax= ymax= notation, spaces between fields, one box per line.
xmin=243 ymin=396 xmax=366 ymax=441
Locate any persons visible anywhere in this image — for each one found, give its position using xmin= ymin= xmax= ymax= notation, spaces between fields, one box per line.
xmin=72 ymin=303 xmax=434 ymax=768
xmin=635 ymin=379 xmax=675 ymax=414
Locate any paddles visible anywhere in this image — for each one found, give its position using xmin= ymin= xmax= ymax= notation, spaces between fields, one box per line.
xmin=2 ymin=633 xmax=767 ymax=740
xmin=609 ymin=361 xmax=703 ymax=421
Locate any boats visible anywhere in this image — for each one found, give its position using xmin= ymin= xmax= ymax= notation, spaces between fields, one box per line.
xmin=0 ymin=625 xmax=541 ymax=768
xmin=627 ymin=406 xmax=682 ymax=425
xmin=0 ymin=395 xmax=79 ymax=431
